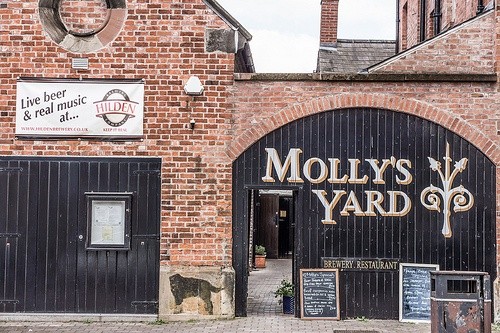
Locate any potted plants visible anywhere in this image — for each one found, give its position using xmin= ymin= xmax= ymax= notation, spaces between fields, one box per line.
xmin=274 ymin=280 xmax=295 ymax=314
xmin=256 ymin=245 xmax=266 ymax=267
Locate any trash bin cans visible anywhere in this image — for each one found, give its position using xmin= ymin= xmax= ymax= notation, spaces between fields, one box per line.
xmin=430 ymin=268 xmax=490 ymax=332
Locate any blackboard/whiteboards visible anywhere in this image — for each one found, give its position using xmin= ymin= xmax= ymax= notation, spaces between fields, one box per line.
xmin=398 ymin=263 xmax=437 ymax=320
xmin=300 ymin=269 xmax=340 ymax=319
